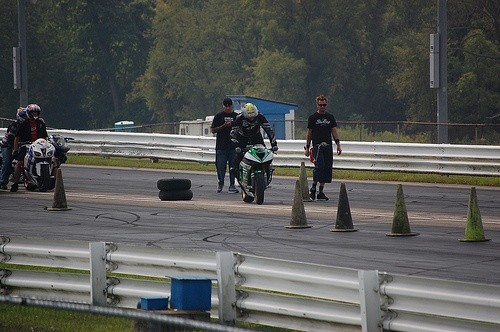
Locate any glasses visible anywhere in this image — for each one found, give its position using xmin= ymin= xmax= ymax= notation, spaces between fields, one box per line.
xmin=317 ymin=102 xmax=327 ymax=106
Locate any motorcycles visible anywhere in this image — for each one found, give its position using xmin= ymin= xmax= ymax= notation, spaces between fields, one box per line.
xmin=0 ymin=133 xmax=75 ymax=193
xmin=234 ymin=143 xmax=279 ymax=206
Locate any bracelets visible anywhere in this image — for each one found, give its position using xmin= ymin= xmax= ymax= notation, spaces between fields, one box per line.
xmin=304 ymin=145 xmax=309 ymax=150
xmin=335 ymin=139 xmax=339 ymax=143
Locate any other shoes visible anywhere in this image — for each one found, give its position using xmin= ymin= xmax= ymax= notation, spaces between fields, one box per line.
xmin=228 ymin=186 xmax=239 ymax=193
xmin=0 ymin=182 xmax=10 ymax=191
xmin=10 ymin=184 xmax=19 ymax=193
xmin=317 ymin=192 xmax=328 ymax=201
xmin=309 ymin=187 xmax=316 ymax=200
xmin=216 ymin=182 xmax=224 ymax=192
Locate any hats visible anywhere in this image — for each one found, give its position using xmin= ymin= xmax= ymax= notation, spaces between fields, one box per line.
xmin=223 ymin=98 xmax=232 ymax=106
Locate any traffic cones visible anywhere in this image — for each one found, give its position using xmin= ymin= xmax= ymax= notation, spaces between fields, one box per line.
xmin=47 ymin=168 xmax=73 ymax=211
xmin=298 ymin=162 xmax=314 ymax=202
xmin=329 ymin=182 xmax=359 ymax=232
xmin=458 ymin=187 xmax=492 ymax=242
xmin=385 ymin=184 xmax=420 ymax=236
xmin=284 ymin=179 xmax=314 ymax=229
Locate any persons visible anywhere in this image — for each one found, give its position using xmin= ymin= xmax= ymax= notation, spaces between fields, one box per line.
xmin=210 ymin=98 xmax=239 ymax=194
xmin=304 ymin=95 xmax=343 ymax=200
xmin=0 ymin=103 xmax=50 ymax=192
xmin=230 ymin=102 xmax=279 ymax=188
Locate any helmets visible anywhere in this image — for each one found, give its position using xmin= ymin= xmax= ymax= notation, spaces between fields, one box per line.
xmin=16 ymin=107 xmax=26 ymax=122
xmin=242 ymin=102 xmax=258 ymax=120
xmin=26 ymin=104 xmax=41 ymax=120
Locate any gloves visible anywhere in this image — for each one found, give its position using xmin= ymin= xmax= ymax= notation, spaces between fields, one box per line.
xmin=234 ymin=146 xmax=241 ymax=153
xmin=272 ymin=145 xmax=278 ymax=151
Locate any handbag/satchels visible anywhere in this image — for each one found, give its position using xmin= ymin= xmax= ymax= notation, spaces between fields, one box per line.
xmin=310 ymin=148 xmax=316 ymax=163
